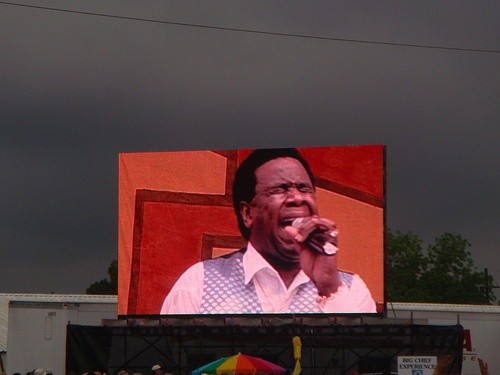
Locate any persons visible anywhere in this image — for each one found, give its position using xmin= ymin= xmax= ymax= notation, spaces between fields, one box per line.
xmin=433 ymin=355 xmax=489 ymax=375
xmin=161 ymin=147 xmax=378 ymax=315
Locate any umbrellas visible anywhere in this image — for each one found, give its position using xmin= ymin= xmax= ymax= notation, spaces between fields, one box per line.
xmin=192 ymin=352 xmax=285 ymax=375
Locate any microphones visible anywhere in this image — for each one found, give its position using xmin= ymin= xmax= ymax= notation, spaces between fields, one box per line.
xmin=291 ymin=217 xmax=338 ymax=256
